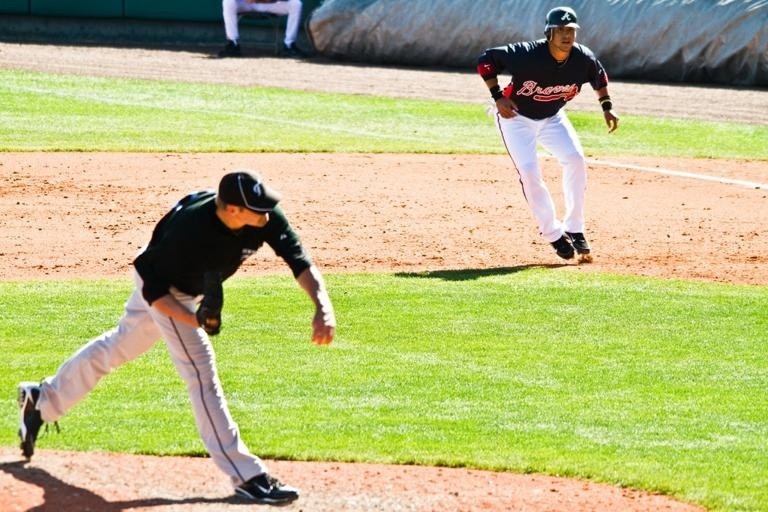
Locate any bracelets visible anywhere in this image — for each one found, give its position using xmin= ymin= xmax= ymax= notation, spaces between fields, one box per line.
xmin=489 ymin=85 xmax=504 ymax=102
xmin=598 ymin=95 xmax=613 ymax=112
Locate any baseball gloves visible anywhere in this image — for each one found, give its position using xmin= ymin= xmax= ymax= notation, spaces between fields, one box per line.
xmin=196 ymin=270 xmax=222 ymax=334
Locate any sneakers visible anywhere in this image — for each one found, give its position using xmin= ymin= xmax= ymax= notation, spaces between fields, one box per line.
xmin=16 ymin=381 xmax=44 ymax=460
xmin=549 ymin=231 xmax=575 ymax=259
xmin=284 ymin=42 xmax=307 ymax=57
xmin=218 ymin=41 xmax=241 ymax=57
xmin=564 ymin=231 xmax=594 ymax=255
xmin=232 ymin=473 xmax=300 ymax=505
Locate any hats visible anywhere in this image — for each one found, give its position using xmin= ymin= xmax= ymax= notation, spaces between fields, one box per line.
xmin=218 ymin=171 xmax=280 ymax=216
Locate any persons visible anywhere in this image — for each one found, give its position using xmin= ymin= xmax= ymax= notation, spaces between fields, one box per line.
xmin=15 ymin=169 xmax=336 ymax=505
xmin=215 ymin=0 xmax=309 ymax=59
xmin=476 ymin=6 xmax=621 ymax=261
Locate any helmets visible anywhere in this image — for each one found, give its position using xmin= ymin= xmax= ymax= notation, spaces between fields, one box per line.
xmin=543 ymin=7 xmax=581 ymax=42
